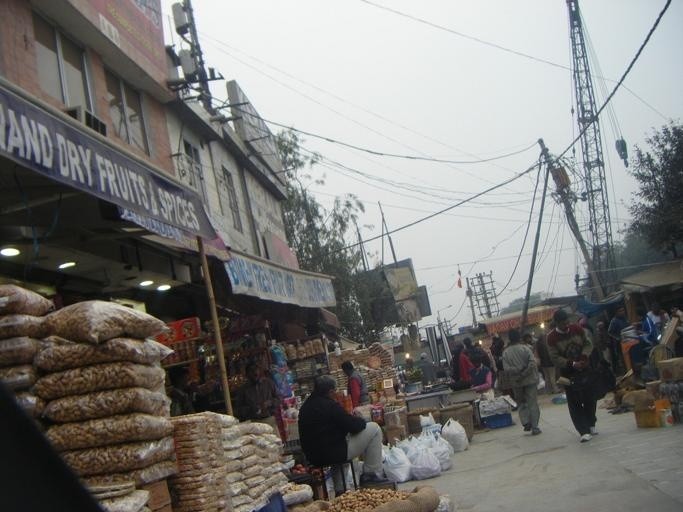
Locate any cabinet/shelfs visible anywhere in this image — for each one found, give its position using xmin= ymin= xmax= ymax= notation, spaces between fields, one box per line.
xmin=159 ymin=336 xmax=201 ymax=367
xmin=283 ymin=333 xmax=331 ymax=394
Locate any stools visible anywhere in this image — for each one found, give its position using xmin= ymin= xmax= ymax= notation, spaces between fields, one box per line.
xmin=309 ymin=460 xmax=358 ymax=501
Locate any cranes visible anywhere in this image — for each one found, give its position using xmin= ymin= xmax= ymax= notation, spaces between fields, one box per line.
xmin=565 ymin=0 xmax=628 ymax=300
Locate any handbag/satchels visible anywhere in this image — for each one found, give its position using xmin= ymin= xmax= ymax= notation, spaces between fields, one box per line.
xmin=573 ymin=359 xmax=617 ymax=403
xmin=381 ymin=412 xmax=469 ymax=484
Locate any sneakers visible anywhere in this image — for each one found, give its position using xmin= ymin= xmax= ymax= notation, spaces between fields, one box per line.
xmin=523 ymin=424 xmax=542 ymax=435
xmin=580 ymin=433 xmax=592 ymax=442
xmin=590 ymin=426 xmax=599 ymax=434
xmin=359 ymin=472 xmax=390 ymax=484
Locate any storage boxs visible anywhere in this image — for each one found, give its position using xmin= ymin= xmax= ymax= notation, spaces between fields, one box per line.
xmin=636 ymin=355 xmax=682 ymax=429
xmin=485 ymin=413 xmax=514 ymax=430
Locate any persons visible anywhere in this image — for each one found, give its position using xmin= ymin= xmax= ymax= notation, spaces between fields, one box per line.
xmin=543 ymin=308 xmax=599 ymax=443
xmin=297 ymin=374 xmax=389 ymax=499
xmin=572 ymin=299 xmax=682 ymax=383
xmin=166 ymin=363 xmax=213 ymax=416
xmin=416 ymin=351 xmax=434 ymax=383
xmin=449 ymin=325 xmax=562 ymax=399
xmin=239 ymin=361 xmax=283 ymax=421
xmin=501 ymin=326 xmax=544 ymax=435
xmin=341 ymin=359 xmax=372 ymax=413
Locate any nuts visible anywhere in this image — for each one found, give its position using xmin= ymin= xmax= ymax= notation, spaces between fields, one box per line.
xmin=0 ymin=283 xmax=412 ymax=512
